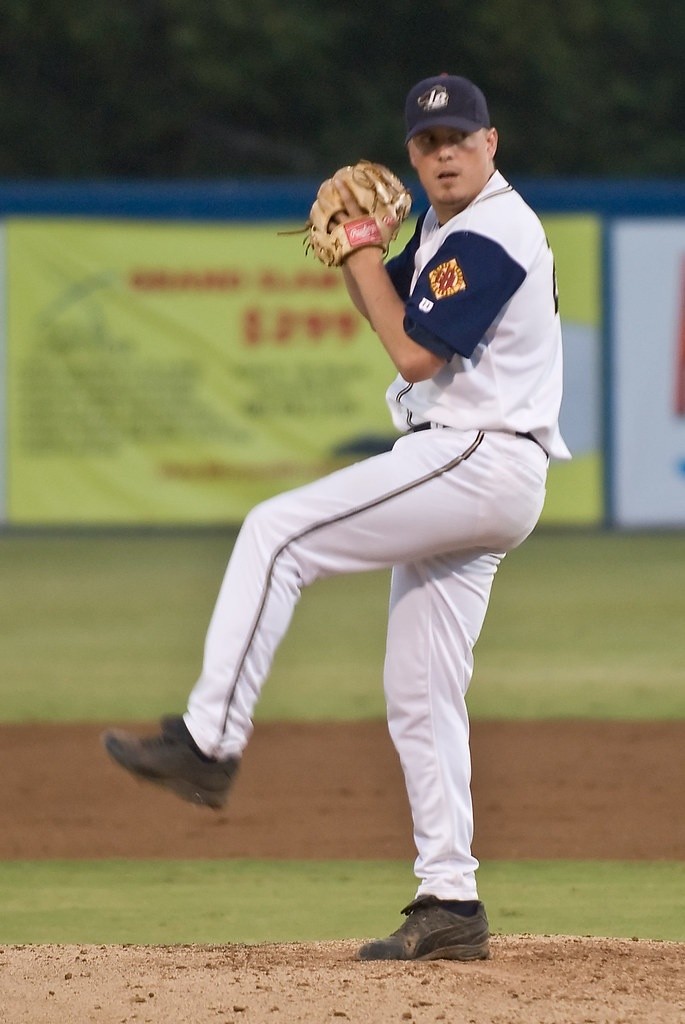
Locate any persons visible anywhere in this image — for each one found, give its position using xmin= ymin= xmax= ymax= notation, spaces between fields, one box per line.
xmin=101 ymin=74 xmax=571 ymax=959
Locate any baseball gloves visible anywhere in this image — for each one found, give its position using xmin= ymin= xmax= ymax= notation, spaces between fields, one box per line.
xmin=306 ymin=158 xmax=413 ymax=269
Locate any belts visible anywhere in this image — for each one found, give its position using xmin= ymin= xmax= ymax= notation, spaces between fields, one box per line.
xmin=407 ymin=421 xmax=550 ymax=457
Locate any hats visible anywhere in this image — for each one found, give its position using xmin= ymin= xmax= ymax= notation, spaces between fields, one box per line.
xmin=404 ymin=72 xmax=491 ymax=145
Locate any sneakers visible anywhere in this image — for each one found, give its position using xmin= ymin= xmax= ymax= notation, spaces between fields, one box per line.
xmin=356 ymin=893 xmax=491 ymax=962
xmin=104 ymin=715 xmax=237 ymax=810
